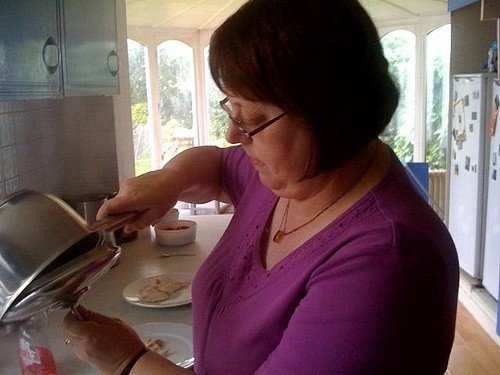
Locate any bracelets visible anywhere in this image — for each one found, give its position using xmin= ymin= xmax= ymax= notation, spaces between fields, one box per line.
xmin=120 ymin=346 xmax=150 ymax=375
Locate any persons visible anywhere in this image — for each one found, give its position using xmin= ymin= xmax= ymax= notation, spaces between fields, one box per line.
xmin=61 ymin=0 xmax=459 ymax=375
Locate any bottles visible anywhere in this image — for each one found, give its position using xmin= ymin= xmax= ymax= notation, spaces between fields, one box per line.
xmin=487 ymin=47 xmax=496 ymax=71
xmin=18 ymin=312 xmax=57 ymax=375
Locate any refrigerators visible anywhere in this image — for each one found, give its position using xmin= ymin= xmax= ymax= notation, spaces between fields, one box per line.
xmin=447 ymin=71 xmax=500 ymax=303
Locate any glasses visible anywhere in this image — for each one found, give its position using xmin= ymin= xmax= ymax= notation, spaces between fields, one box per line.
xmin=218 ymin=96 xmax=290 ymax=141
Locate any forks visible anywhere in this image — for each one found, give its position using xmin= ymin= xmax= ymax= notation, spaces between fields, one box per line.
xmin=159 ymin=250 xmax=196 ymax=257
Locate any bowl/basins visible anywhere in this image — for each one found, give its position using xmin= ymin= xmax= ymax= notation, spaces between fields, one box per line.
xmin=154 ymin=218 xmax=197 ymax=246
xmin=155 ymin=208 xmax=179 ymax=227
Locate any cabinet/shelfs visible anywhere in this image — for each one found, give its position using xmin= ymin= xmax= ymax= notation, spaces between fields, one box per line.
xmin=0 ymin=0 xmax=129 ymax=100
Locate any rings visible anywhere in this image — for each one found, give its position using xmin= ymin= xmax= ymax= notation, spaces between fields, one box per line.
xmin=64 ymin=338 xmax=71 ymax=346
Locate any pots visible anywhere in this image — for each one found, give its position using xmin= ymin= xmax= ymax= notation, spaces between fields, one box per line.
xmin=1 ymin=188 xmax=144 ymax=324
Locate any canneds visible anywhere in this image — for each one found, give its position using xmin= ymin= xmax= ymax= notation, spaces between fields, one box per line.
xmin=487 ymin=48 xmax=497 ymax=72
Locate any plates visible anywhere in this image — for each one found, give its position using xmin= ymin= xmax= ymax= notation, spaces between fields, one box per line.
xmin=121 ymin=270 xmax=194 ymax=308
xmin=135 ymin=321 xmax=194 ymax=370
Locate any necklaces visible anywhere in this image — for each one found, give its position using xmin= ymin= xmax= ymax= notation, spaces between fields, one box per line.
xmin=273 ymin=139 xmax=381 ymax=243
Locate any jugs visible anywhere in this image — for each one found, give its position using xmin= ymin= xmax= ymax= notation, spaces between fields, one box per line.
xmin=72 ymin=193 xmax=120 ymax=268
